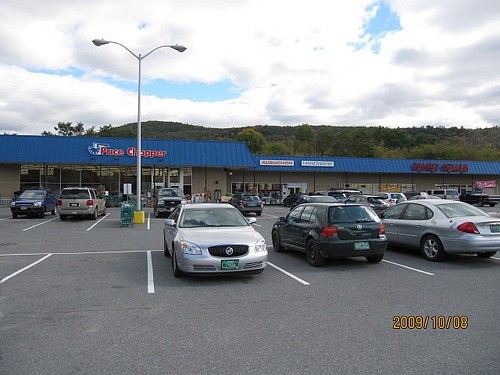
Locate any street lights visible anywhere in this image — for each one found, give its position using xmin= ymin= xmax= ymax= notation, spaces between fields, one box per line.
xmin=91 ymin=37 xmax=187 ymax=224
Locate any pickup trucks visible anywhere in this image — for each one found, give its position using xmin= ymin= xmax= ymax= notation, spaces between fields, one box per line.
xmin=9 ymin=189 xmax=58 ymax=219
xmin=459 ymin=191 xmax=500 ymax=207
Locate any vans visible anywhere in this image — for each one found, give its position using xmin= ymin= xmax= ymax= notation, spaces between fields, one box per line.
xmin=419 ymin=189 xmax=460 ymax=201
xmin=152 ymin=188 xmax=186 ymax=217
xmin=56 ymin=186 xmax=107 ymax=220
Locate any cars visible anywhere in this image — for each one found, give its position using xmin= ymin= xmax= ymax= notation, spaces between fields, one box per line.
xmin=283 ymin=193 xmax=309 ymax=207
xmin=377 ymin=198 xmax=500 ymax=261
xmin=344 ymin=195 xmax=395 ymax=213
xmin=227 ymin=193 xmax=263 ymax=216
xmin=315 ymin=189 xmax=420 ymax=205
xmin=383 ymin=193 xmax=407 ymax=203
xmin=162 ymin=203 xmax=268 ymax=276
xmin=291 ymin=195 xmax=338 ymax=210
xmin=271 ymin=203 xmax=387 ymax=268
